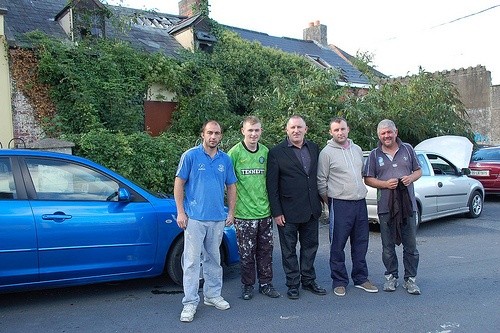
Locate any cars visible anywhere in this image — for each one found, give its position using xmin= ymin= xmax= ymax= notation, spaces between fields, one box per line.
xmin=0 ymin=148 xmax=241 ymax=293
xmin=324 ymin=135 xmax=485 ymax=234
xmin=469 ymin=146 xmax=500 ymax=199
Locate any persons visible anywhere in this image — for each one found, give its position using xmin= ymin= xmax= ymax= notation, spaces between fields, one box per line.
xmin=227 ymin=115 xmax=281 ymax=300
xmin=267 ymin=115 xmax=327 ymax=299
xmin=362 ymin=119 xmax=423 ymax=294
xmin=173 ymin=121 xmax=238 ymax=321
xmin=317 ymin=117 xmax=378 ymax=296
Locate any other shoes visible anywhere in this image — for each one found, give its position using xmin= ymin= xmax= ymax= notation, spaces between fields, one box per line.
xmin=333 ymin=285 xmax=347 ymax=297
xmin=354 ymin=280 xmax=379 ymax=293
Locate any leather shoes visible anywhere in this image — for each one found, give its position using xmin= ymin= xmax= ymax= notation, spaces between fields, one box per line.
xmin=286 ymin=285 xmax=300 ymax=299
xmin=302 ymin=279 xmax=327 ymax=295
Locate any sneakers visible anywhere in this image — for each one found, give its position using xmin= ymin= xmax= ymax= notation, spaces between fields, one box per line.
xmin=402 ymin=276 xmax=421 ymax=295
xmin=203 ymin=295 xmax=231 ymax=310
xmin=241 ymin=283 xmax=256 ymax=300
xmin=382 ymin=273 xmax=400 ymax=292
xmin=258 ymin=282 xmax=281 ymax=298
xmin=180 ymin=303 xmax=198 ymax=322
